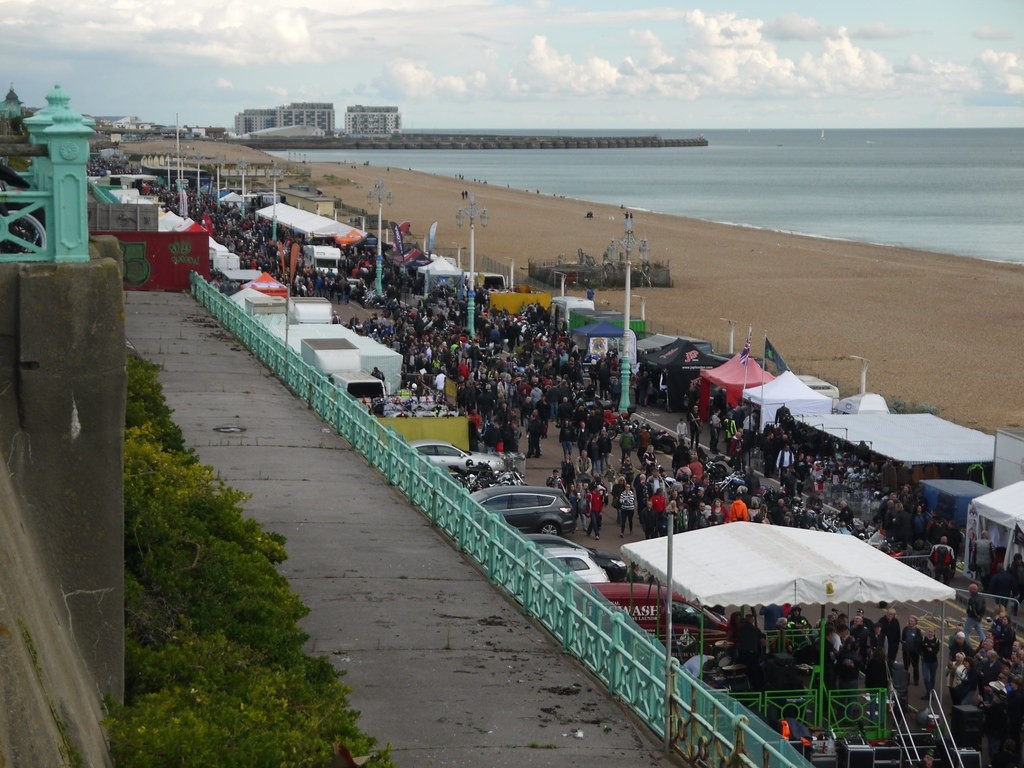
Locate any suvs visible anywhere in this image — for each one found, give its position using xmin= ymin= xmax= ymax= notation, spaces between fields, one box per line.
xmin=518 ymin=545 xmax=610 ymax=592
xmin=469 ymin=486 xmax=578 ymax=536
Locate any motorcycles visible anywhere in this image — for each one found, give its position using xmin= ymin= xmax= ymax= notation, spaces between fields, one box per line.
xmin=602 ymin=403 xmax=678 ymax=455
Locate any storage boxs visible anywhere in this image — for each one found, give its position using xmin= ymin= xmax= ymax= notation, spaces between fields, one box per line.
xmin=838 ymin=740 xmax=902 ymax=768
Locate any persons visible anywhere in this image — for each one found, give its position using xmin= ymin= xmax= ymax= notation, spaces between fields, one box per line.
xmin=546 ymin=398 xmax=1024 ymax=768
xmin=86 ymin=155 xmax=140 ymax=177
xmin=217 ymin=208 xmax=425 ymax=305
xmin=138 ymin=181 xmax=240 ymax=244
xmin=332 ymin=279 xmax=666 ymax=459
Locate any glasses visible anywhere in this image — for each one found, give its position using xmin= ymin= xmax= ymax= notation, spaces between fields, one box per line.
xmin=699 ymin=505 xmax=706 ymax=508
xmin=620 ymin=480 xmax=625 ymax=482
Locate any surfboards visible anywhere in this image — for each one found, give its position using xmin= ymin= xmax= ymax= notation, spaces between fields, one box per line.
xmin=153 ymin=155 xmax=159 ymax=167
xmin=147 ymin=155 xmax=153 ymax=167
xmin=141 ymin=155 xmax=148 ymax=166
xmin=160 ymin=155 xmax=165 ymax=166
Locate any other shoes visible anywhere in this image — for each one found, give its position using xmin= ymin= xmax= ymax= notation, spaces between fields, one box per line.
xmin=914 ymin=681 xmax=919 ymax=685
xmin=596 ymin=535 xmax=599 ymax=539
xmin=584 ymin=527 xmax=587 ymax=531
xmin=619 ymin=534 xmax=624 ymax=538
xmin=923 ymin=695 xmax=933 ymax=700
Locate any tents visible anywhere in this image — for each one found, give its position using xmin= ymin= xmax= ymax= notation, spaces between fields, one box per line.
xmin=636 ymin=333 xmax=890 ymax=435
xmin=255 ymin=202 xmax=463 ymax=294
xmin=964 ymin=481 xmax=1024 ymax=578
xmin=791 ymin=413 xmax=995 ymax=464
xmin=571 ymin=320 xmax=624 ymax=354
xmin=201 ymin=181 xmax=283 ymax=207
xmin=109 ymin=189 xmax=262 ymax=293
xmin=550 ymin=296 xmax=595 ymax=334
xmin=220 ymin=192 xmax=242 ymax=202
xmin=229 ymin=272 xmax=403 ymax=395
xmin=919 ymin=479 xmax=995 ymax=529
xmin=620 ymin=521 xmax=956 ymax=744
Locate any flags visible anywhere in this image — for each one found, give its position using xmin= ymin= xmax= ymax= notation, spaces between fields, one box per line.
xmin=764 ymin=336 xmax=790 ymax=373
xmin=739 ymin=326 xmax=752 ymax=369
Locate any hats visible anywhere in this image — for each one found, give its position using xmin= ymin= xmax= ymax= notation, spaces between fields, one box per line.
xmin=957 ymin=631 xmax=966 ymax=638
xmin=989 ymin=682 xmax=1008 ymax=693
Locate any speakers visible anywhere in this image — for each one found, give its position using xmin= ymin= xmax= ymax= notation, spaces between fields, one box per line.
xmin=950 ymin=705 xmax=983 ymax=747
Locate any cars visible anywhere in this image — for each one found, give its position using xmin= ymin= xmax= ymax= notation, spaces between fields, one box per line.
xmin=406 ymin=440 xmax=504 ymax=475
xmin=521 ymin=532 xmax=629 ymax=581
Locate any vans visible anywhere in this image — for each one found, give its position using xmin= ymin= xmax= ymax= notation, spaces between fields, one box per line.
xmin=572 ymin=581 xmax=729 ymax=664
xmin=332 ymin=371 xmax=386 ymax=412
xmin=302 ymin=244 xmax=341 ymax=277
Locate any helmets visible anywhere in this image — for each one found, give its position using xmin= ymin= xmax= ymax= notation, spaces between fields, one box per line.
xmin=969 ymin=584 xmax=979 ymax=592
